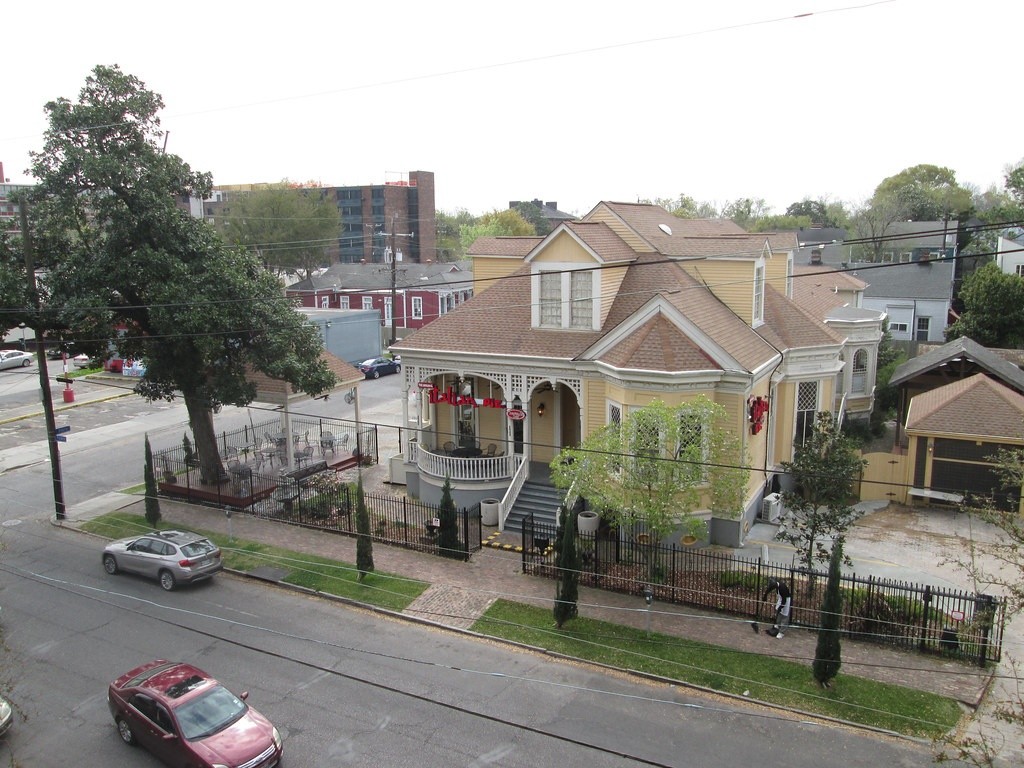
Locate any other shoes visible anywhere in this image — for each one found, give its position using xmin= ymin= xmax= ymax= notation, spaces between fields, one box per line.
xmin=776 ymin=632 xmax=785 ymax=639
xmin=774 ymin=624 xmax=780 ymax=630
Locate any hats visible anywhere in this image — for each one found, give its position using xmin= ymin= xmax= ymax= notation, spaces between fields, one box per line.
xmin=768 ymin=579 xmax=776 ymax=587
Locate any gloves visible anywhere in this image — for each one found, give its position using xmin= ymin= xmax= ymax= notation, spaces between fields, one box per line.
xmin=762 ymin=594 xmax=767 ymax=601
xmin=777 ymin=607 xmax=782 ymax=612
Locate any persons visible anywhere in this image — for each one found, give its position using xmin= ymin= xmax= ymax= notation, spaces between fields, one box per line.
xmin=764 ymin=581 xmax=791 ymax=639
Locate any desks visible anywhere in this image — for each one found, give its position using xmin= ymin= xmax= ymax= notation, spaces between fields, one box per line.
xmin=261 ymin=447 xmax=280 ymax=466
xmin=907 ymin=488 xmax=964 ymax=520
xmin=321 ymin=436 xmax=337 ymax=454
xmin=451 ymin=448 xmax=483 ymax=458
xmin=234 ymin=442 xmax=255 ymax=458
xmin=274 ymin=432 xmax=287 ymax=447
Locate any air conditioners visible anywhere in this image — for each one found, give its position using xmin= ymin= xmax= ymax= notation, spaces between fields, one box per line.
xmin=761 ymin=493 xmax=781 ymax=522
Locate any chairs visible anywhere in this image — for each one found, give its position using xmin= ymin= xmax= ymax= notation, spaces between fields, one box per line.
xmin=470 ymin=441 xmax=481 ymax=458
xmin=219 ymin=427 xmax=349 ymax=486
xmin=493 ymin=451 xmax=505 ymax=467
xmin=483 ymin=444 xmax=497 ymax=456
xmin=443 ymin=441 xmax=456 ymax=456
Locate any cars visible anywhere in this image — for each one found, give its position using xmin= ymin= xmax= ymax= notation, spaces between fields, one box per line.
xmin=0 ymin=349 xmax=35 ymax=370
xmin=105 ymin=658 xmax=283 ymax=768
xmin=359 ymin=357 xmax=400 ymax=378
xmin=46 ymin=343 xmax=80 ymax=359
xmin=73 ymin=353 xmax=96 ymax=369
xmin=0 ymin=696 xmax=14 ymax=738
xmin=101 ymin=528 xmax=224 ymax=592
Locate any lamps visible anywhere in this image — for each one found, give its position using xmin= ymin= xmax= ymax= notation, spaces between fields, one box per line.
xmin=927 ymin=442 xmax=934 ymax=456
xmin=537 ymin=403 xmax=545 ymax=418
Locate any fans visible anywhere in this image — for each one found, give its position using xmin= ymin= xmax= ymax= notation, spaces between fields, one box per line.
xmin=447 ymin=377 xmax=472 ymax=385
xmin=486 ymin=383 xmax=501 ymax=390
xmin=534 ymin=381 xmax=560 ymax=394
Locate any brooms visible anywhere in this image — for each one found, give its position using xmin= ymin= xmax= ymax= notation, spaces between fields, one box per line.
xmin=751 ymin=606 xmax=773 ymax=635
